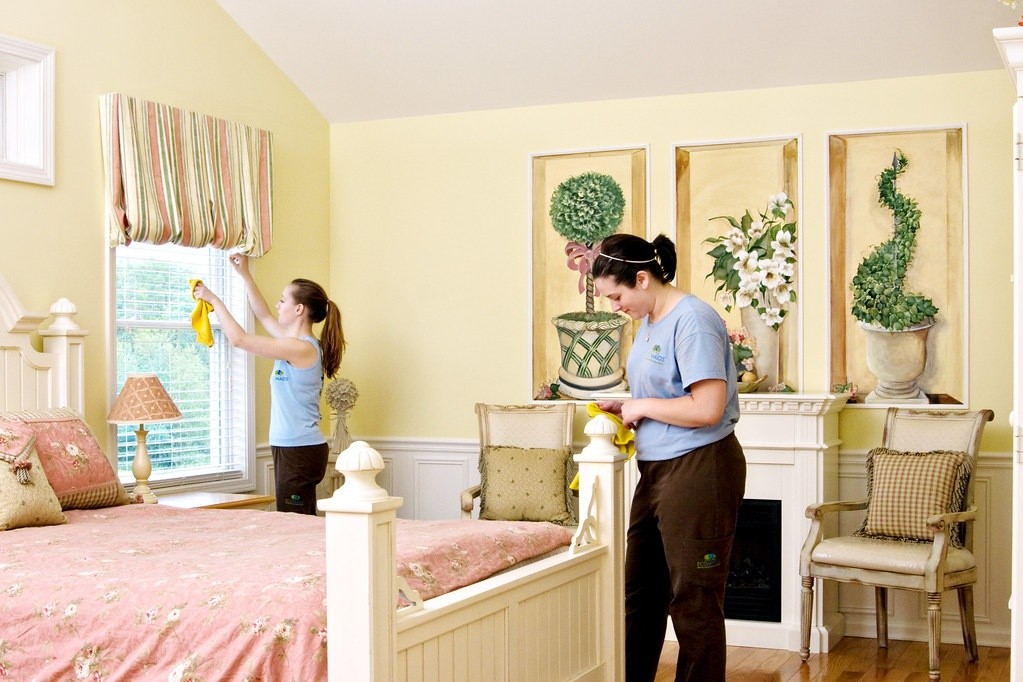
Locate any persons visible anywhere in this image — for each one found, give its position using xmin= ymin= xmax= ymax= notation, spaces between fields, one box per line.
xmin=592 ymin=233 xmax=746 ymax=682
xmin=194 ymin=252 xmax=349 ymax=516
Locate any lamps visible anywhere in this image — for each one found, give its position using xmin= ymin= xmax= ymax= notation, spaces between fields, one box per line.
xmin=104 ymin=373 xmax=183 ymax=504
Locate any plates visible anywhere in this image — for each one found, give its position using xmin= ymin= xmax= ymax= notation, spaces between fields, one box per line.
xmin=737 ymin=375 xmax=770 ymax=393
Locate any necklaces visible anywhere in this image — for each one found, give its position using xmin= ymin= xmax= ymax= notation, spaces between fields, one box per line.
xmin=646 ymin=317 xmax=651 ymax=342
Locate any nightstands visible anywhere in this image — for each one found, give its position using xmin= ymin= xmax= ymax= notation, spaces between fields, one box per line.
xmin=151 ymin=487 xmax=277 ymax=510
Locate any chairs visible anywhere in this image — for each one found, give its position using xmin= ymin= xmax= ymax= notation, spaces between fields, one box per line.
xmin=799 ymin=407 xmax=994 ymax=682
xmin=460 ymin=401 xmax=581 ymax=530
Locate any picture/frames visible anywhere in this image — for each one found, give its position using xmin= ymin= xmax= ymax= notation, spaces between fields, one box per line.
xmin=525 ymin=144 xmax=654 ymax=403
xmin=666 ymin=133 xmax=804 ymax=399
xmin=0 ymin=35 xmax=56 ymax=187
xmin=822 ymin=122 xmax=970 ymax=415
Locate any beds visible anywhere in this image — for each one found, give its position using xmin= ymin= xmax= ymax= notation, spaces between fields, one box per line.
xmin=0 ymin=295 xmax=628 ymax=682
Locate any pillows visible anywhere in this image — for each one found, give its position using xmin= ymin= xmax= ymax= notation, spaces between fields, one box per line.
xmin=0 ymin=405 xmax=129 ymax=531
xmin=850 ymin=447 xmax=973 ymax=549
xmin=476 ymin=445 xmax=575 ymax=527
